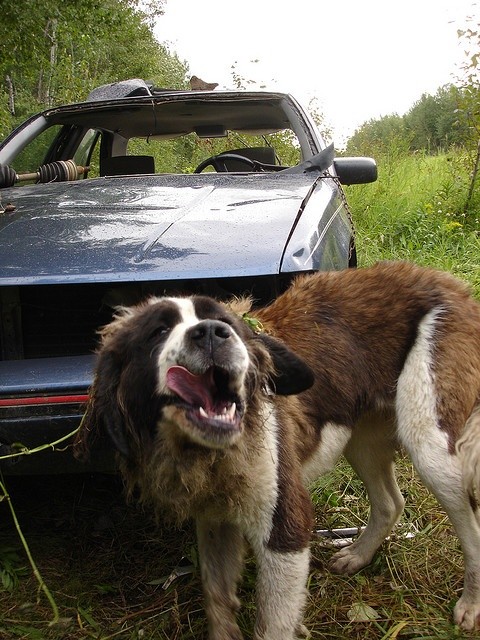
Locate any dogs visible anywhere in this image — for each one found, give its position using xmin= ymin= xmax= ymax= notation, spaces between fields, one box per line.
xmin=73 ymin=258 xmax=479 ymax=640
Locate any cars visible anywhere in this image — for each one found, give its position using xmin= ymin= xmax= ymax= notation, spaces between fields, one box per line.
xmin=1 ymin=75 xmax=377 ymax=477
